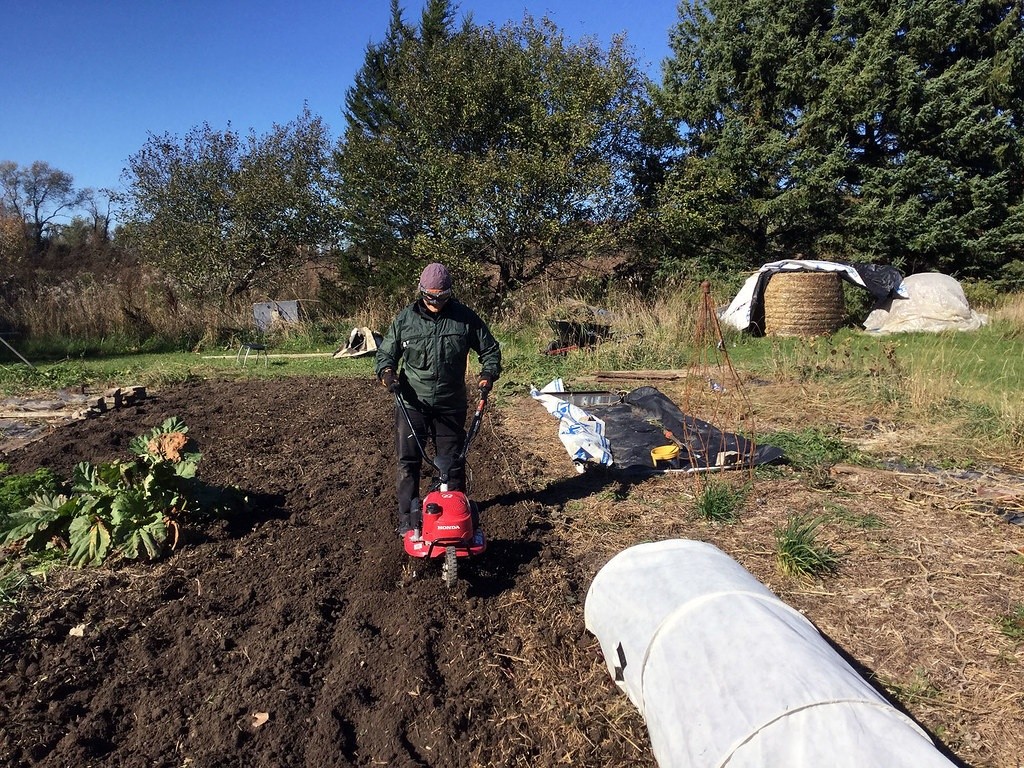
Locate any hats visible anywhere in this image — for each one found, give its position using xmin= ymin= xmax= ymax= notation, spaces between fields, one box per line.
xmin=420 ymin=262 xmax=451 ymax=290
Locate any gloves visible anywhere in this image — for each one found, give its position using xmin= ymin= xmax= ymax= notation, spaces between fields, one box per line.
xmin=382 ymin=370 xmax=400 ymax=393
xmin=477 ymin=375 xmax=493 ymax=392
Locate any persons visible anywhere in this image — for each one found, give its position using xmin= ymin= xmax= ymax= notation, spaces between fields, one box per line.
xmin=375 ymin=262 xmax=503 ymax=538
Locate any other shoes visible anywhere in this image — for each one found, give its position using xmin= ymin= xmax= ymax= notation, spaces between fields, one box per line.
xmin=397 ymin=520 xmax=411 ymax=537
xmin=472 ymin=529 xmax=485 ymax=546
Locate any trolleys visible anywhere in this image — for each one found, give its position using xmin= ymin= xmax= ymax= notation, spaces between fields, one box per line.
xmin=391 ymin=384 xmax=488 ymax=590
